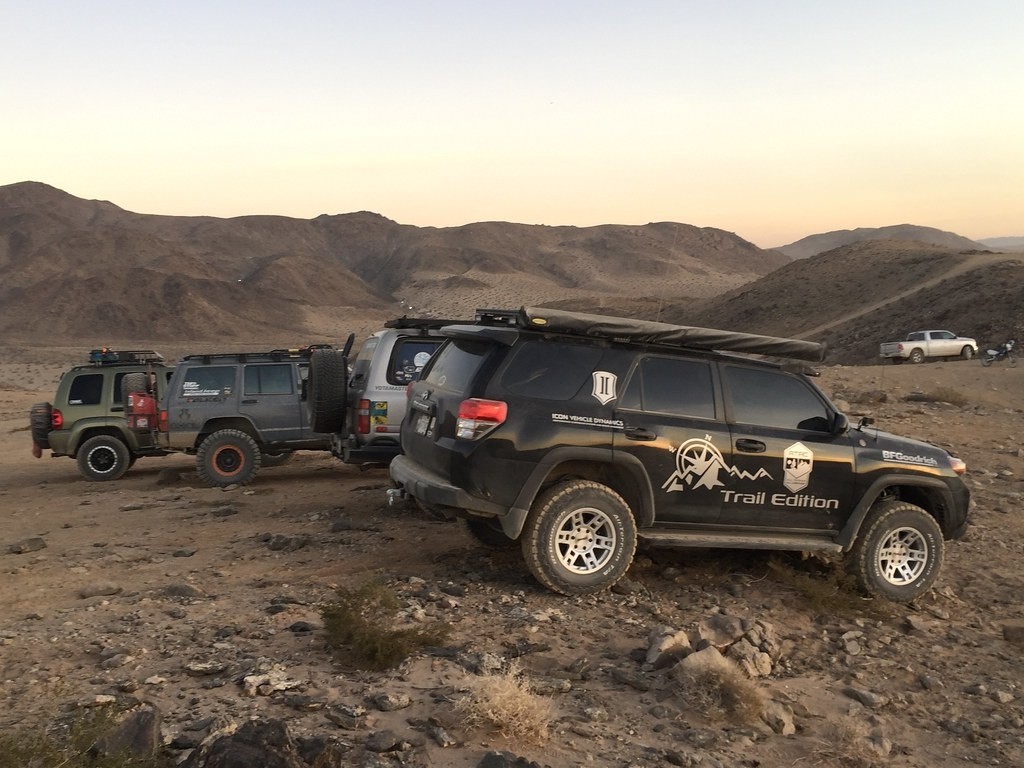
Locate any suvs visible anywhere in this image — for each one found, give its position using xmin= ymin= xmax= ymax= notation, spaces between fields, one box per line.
xmin=300 ymin=316 xmax=476 ymax=474
xmin=120 ymin=342 xmax=344 ymax=487
xmin=385 ymin=305 xmax=971 ymax=601
xmin=31 ymin=349 xmax=183 ymax=481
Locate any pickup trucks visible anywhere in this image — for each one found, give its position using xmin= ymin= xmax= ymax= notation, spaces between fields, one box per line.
xmin=879 ymin=330 xmax=979 ymax=364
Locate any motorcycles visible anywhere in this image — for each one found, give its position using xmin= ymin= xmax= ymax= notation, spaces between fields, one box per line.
xmin=979 ymin=339 xmax=1019 ymax=369
